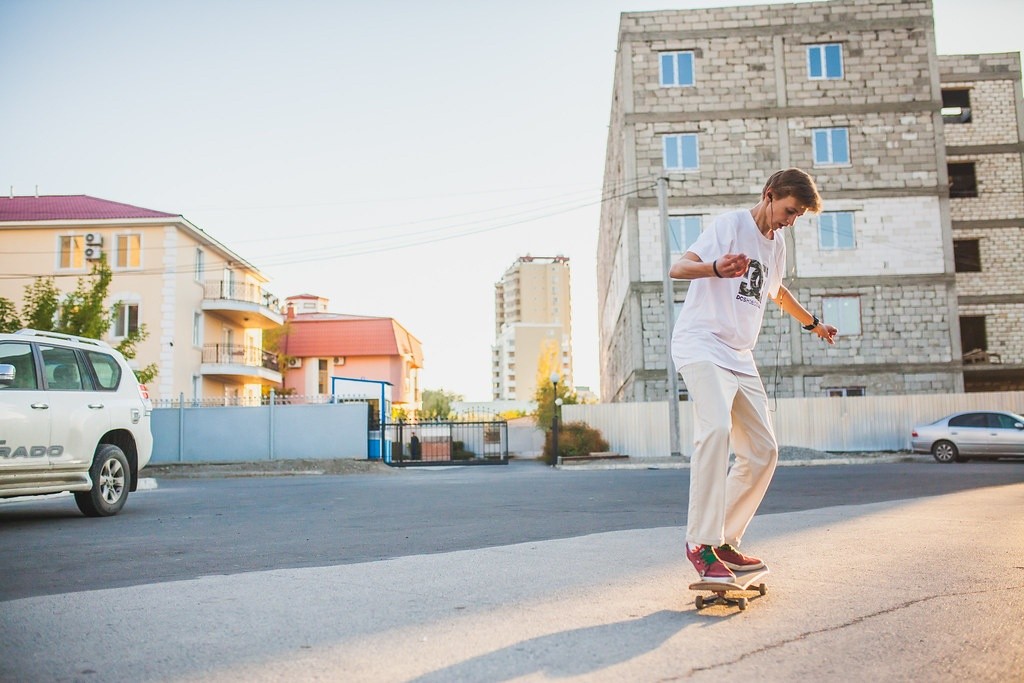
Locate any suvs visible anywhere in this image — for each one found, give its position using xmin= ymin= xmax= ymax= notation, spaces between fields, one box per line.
xmin=0 ymin=329 xmax=153 ymax=518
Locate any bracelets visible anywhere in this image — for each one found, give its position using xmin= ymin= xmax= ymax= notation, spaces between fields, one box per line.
xmin=713 ymin=260 xmax=723 ymax=278
xmin=803 ymin=316 xmax=819 ymax=330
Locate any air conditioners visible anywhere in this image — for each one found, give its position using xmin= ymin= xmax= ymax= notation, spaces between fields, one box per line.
xmin=332 ymin=356 xmax=345 ymax=365
xmin=84 ymin=232 xmax=103 ymax=246
xmin=83 ymin=245 xmax=101 ymax=259
xmin=288 ymin=358 xmax=301 ymax=369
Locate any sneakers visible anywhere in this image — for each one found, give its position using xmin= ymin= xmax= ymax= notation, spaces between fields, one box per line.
xmin=686 ymin=541 xmax=737 ymax=583
xmin=713 ymin=543 xmax=765 ymax=572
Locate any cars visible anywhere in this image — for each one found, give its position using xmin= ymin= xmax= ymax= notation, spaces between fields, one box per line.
xmin=911 ymin=410 xmax=1024 ymax=464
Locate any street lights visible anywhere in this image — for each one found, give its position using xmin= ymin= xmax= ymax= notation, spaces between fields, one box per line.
xmin=551 ymin=373 xmax=558 ymax=468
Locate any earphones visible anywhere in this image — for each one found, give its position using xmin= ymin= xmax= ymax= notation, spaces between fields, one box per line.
xmin=768 ymin=192 xmax=773 ymax=202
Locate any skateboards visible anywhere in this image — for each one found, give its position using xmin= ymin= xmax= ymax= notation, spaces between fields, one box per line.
xmin=687 ymin=564 xmax=771 ymax=610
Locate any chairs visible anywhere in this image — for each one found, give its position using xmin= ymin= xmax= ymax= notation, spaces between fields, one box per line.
xmin=49 ymin=364 xmax=80 ymax=389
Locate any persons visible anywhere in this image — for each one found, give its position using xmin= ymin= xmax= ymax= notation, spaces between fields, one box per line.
xmin=669 ymin=169 xmax=837 ymax=583
xmin=411 ymin=432 xmax=419 ymax=460
